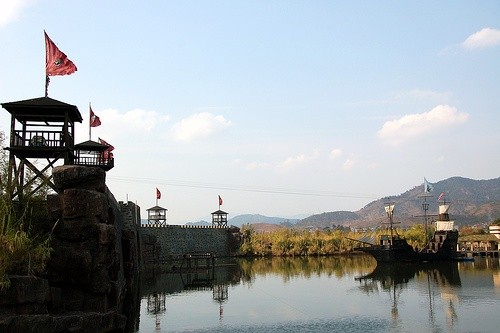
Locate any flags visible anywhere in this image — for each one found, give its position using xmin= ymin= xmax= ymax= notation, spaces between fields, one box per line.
xmin=437 ymin=191 xmax=451 ymax=203
xmin=97 ymin=137 xmax=115 ymax=161
xmin=156 ymin=187 xmax=161 ymax=199
xmin=44 ymin=29 xmax=78 ymax=78
xmin=90 ymin=106 xmax=102 ymax=129
xmin=219 ymin=195 xmax=223 ymax=205
xmin=423 ymin=176 xmax=434 ymax=194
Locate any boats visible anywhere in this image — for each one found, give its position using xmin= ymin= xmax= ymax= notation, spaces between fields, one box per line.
xmin=343 ymin=192 xmax=459 ymax=265
xmin=357 ymin=259 xmax=461 ymax=328
